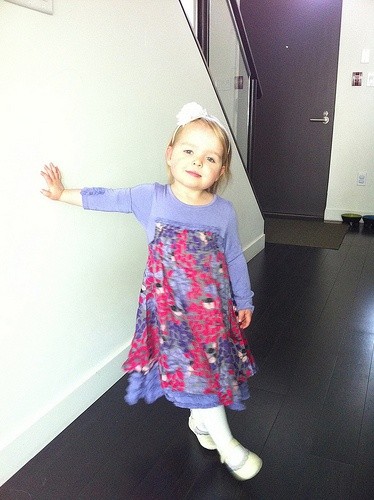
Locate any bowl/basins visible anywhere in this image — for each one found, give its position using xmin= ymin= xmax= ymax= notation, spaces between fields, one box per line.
xmin=362 ymin=215 xmax=374 ymax=225
xmin=341 ymin=213 xmax=362 ymax=223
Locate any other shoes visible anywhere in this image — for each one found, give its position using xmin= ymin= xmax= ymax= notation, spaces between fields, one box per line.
xmin=189 ymin=415 xmax=217 ymax=450
xmin=216 ymin=436 xmax=263 ymax=481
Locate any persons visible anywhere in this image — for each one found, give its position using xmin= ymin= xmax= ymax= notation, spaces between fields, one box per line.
xmin=39 ymin=102 xmax=263 ymax=480
